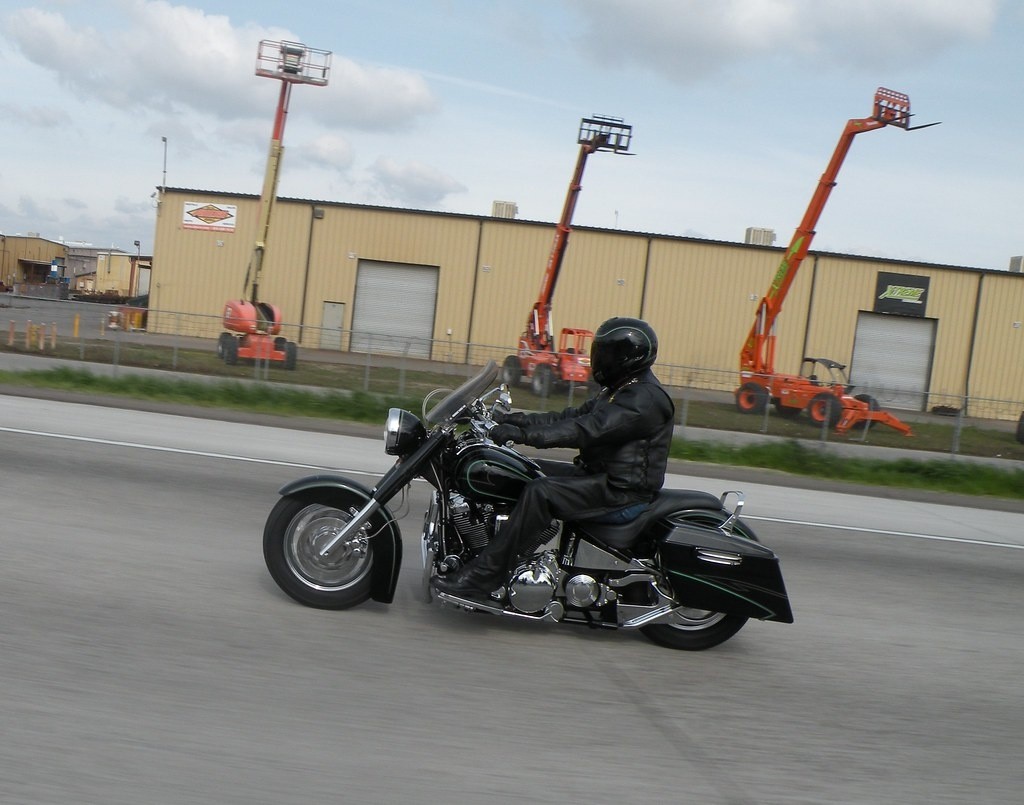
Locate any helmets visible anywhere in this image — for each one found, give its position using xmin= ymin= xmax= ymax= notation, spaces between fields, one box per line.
xmin=590 ymin=317 xmax=658 ymax=385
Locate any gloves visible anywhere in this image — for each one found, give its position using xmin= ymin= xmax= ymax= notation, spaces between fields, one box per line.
xmin=488 ymin=408 xmax=522 ymax=425
xmin=492 ymin=424 xmax=526 ymax=447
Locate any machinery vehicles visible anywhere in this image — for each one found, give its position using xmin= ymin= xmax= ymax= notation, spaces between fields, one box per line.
xmin=217 ymin=39 xmax=333 ymax=370
xmin=727 ymin=87 xmax=943 ymax=436
xmin=490 ymin=112 xmax=637 ymax=399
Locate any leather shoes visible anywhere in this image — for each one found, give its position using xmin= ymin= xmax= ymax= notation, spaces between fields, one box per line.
xmin=429 ymin=571 xmax=488 ymax=605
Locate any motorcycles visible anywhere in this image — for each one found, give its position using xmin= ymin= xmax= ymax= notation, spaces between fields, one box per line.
xmin=261 ymin=357 xmax=794 ymax=651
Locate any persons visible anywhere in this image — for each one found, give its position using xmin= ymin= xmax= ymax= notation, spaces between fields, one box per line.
xmin=429 ymin=316 xmax=676 ymax=604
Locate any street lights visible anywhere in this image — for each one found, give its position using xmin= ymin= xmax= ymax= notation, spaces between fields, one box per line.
xmin=134 ymin=240 xmax=143 ymax=305
